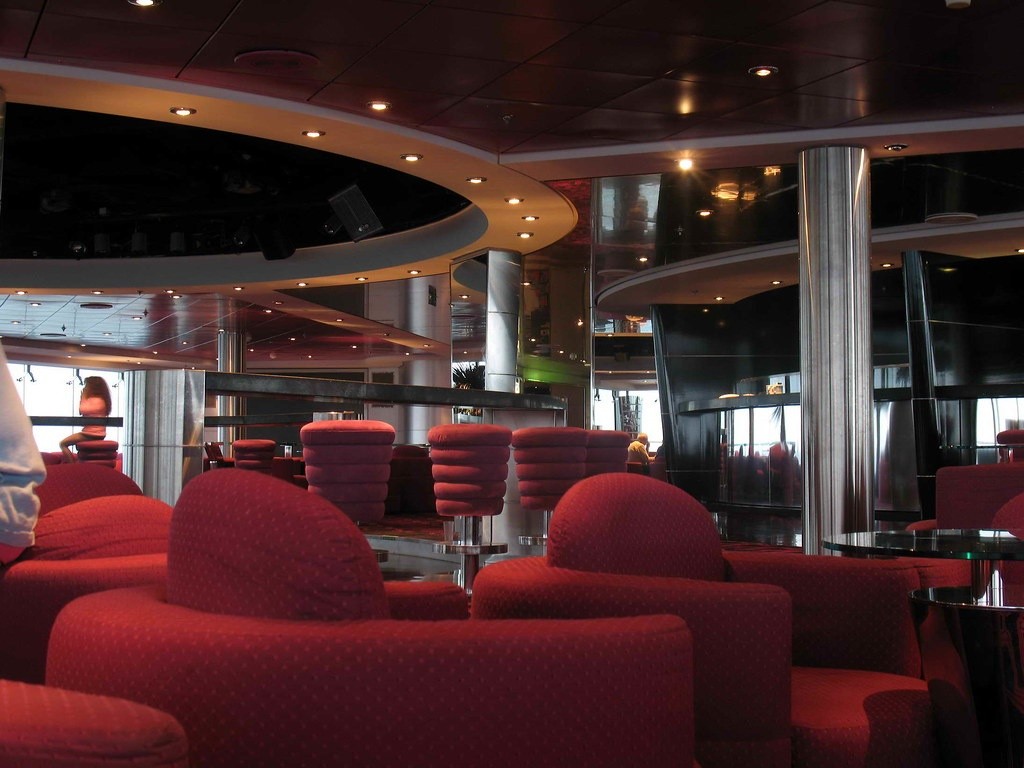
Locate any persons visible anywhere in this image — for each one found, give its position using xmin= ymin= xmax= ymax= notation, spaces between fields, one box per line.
xmin=628 ymin=433 xmax=663 ymax=471
xmin=0 ymin=347 xmax=47 ymax=573
xmin=60 ymin=376 xmax=112 ymax=463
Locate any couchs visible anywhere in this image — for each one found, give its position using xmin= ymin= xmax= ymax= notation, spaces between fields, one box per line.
xmin=0 ymin=443 xmax=1024 ymax=768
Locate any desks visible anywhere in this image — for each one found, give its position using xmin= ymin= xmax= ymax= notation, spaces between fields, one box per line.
xmin=907 ymin=584 xmax=1024 ymax=768
xmin=821 ymin=531 xmax=1024 ymax=561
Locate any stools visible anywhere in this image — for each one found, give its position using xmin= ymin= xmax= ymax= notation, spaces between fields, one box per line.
xmin=231 ymin=439 xmax=277 ymax=476
xmin=301 ymin=421 xmax=396 ymax=532
xmin=997 ymin=430 xmax=1024 ymax=464
xmin=511 ymin=426 xmax=588 ymax=557
xmin=78 ymin=441 xmax=118 ymax=469
xmin=584 ymin=428 xmax=630 ymax=479
xmin=426 ymin=424 xmax=513 ymax=598
